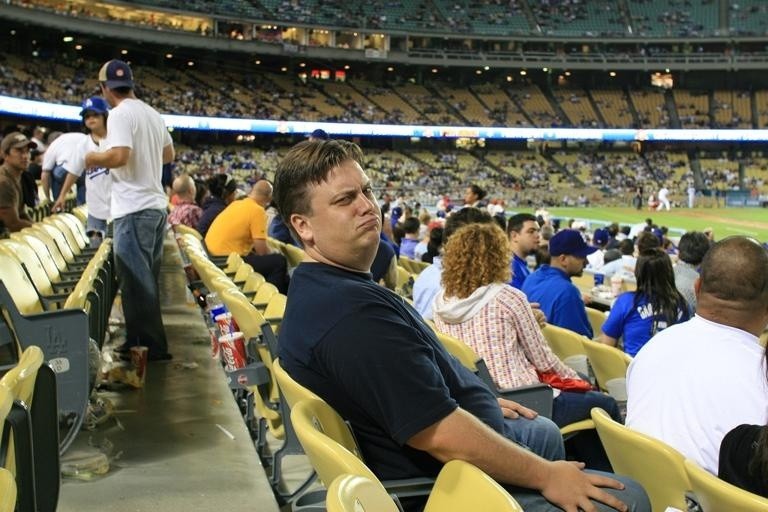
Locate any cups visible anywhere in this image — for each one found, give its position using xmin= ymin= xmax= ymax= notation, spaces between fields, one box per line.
xmin=605 ymin=378 xmax=628 ymax=401
xmin=594 ymin=273 xmax=605 ymax=287
xmin=131 ymin=346 xmax=149 ymax=382
xmin=215 ymin=311 xmax=240 ymax=336
xmin=610 ymin=276 xmax=623 ymax=296
xmin=564 ymin=354 xmax=589 ymax=378
xmin=218 ymin=332 xmax=249 ymax=373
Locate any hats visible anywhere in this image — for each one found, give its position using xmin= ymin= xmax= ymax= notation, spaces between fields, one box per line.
xmin=79 ymin=96 xmax=108 ymax=115
xmin=0 ymin=132 xmax=38 ymax=154
xmin=305 ymin=130 xmax=328 ymax=139
xmin=548 ymin=230 xmax=597 ymax=258
xmin=594 ymin=228 xmax=609 ymax=242
xmin=99 ymin=59 xmax=137 ymax=92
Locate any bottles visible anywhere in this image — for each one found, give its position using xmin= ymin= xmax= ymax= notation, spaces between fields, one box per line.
xmin=206 ymin=292 xmax=229 ymax=324
xmin=185 ymin=285 xmax=206 ymax=309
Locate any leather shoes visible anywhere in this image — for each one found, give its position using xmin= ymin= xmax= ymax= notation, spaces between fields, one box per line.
xmin=113 ymin=340 xmax=138 ymax=354
xmin=119 ymin=350 xmax=167 ymax=362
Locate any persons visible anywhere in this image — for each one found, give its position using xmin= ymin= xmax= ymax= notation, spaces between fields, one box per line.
xmin=371 ymin=1 xmax=768 ymax=359
xmin=0 ymin=0 xmax=369 ymax=248
xmin=205 ymin=180 xmax=290 ymax=296
xmin=431 ymin=222 xmax=622 ymax=474
xmin=85 ymin=60 xmax=175 ymax=361
xmin=625 ymin=234 xmax=768 ymax=477
xmin=272 ymin=139 xmax=653 ymax=512
xmin=717 ymin=338 xmax=768 ymax=500
